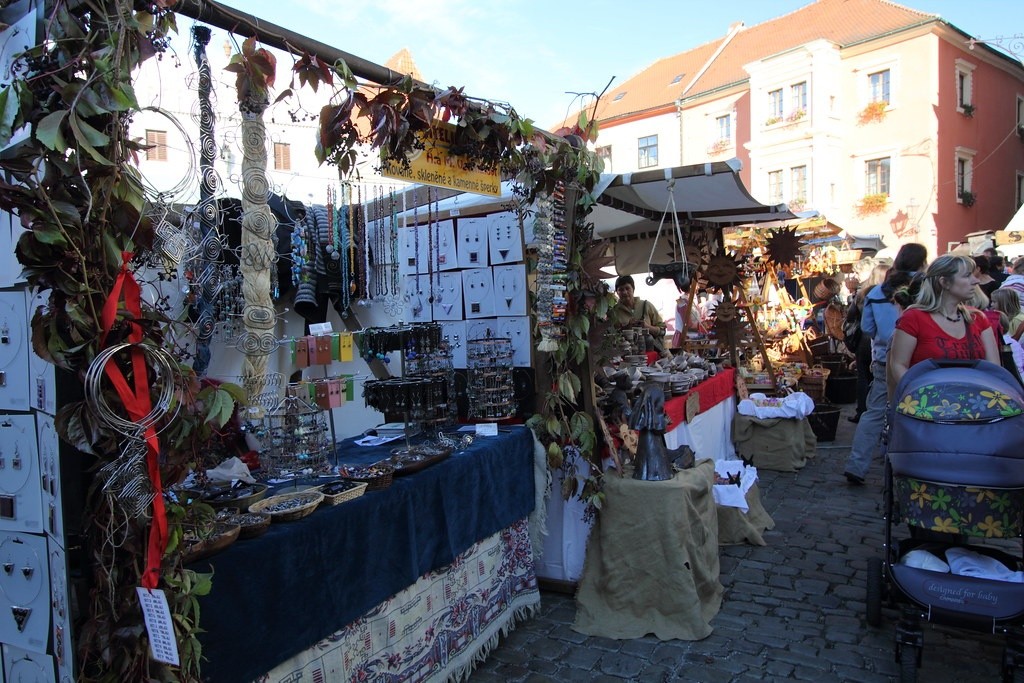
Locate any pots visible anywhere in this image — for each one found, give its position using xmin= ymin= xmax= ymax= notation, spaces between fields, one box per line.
xmin=670 ymin=372 xmax=690 ymax=395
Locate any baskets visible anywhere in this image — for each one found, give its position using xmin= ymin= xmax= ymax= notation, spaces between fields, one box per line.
xmin=226 ymin=508 xmax=271 ymax=539
xmin=172 ymin=488 xmax=203 ymax=506
xmin=395 ymin=446 xmax=452 ymax=477
xmin=836 ymin=242 xmax=862 ymax=265
xmin=185 ymin=523 xmax=241 ymax=561
xmin=247 ymin=492 xmax=324 ymax=521
xmin=823 ymin=302 xmax=845 ymax=341
xmin=346 ymin=464 xmax=396 ymax=491
xmin=302 ymin=480 xmax=369 ymax=507
xmin=801 ymin=364 xmax=831 ymax=385
xmin=206 ymin=481 xmax=268 ymax=513
xmin=846 ymin=270 xmax=859 ymax=290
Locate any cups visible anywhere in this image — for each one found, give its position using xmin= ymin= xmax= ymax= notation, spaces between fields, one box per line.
xmin=625 ymin=354 xmax=648 ymax=368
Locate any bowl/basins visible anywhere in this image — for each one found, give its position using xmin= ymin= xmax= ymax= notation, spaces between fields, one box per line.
xmin=650 ymin=372 xmax=672 ymax=400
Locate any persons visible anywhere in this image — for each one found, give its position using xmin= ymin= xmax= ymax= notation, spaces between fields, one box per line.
xmin=890 ymin=254 xmax=1001 ymax=393
xmin=610 ymin=275 xmax=669 ymax=360
xmin=843 ymin=248 xmax=1024 ymax=425
xmin=843 ymin=244 xmax=929 ymax=485
xmin=671 ymin=277 xmax=703 ymax=348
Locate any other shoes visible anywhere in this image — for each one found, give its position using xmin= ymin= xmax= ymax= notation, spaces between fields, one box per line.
xmin=847 ymin=412 xmax=862 ymax=423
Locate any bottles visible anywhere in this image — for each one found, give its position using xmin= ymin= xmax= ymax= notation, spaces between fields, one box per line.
xmin=620 ymin=327 xmax=654 ymax=359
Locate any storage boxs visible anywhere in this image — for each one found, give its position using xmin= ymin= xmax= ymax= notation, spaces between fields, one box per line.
xmin=801 ymin=336 xmax=860 ymax=442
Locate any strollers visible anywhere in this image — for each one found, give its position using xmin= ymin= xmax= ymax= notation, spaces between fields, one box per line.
xmin=864 ymin=360 xmax=1023 ymax=683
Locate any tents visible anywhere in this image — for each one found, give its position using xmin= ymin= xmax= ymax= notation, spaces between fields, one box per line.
xmin=393 ymin=157 xmax=892 ymax=397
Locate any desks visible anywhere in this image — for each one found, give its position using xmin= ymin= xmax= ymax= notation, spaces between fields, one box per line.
xmin=75 ymin=424 xmax=550 ymax=683
xmin=663 ymin=367 xmax=738 ymax=460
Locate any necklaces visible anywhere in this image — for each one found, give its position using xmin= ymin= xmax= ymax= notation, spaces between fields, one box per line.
xmin=936 ymin=307 xmax=961 ymax=322
xmin=1 ymin=294 xmax=62 ymax=637
xmin=288 ymin=179 xmax=528 ymax=319
xmin=75 ymin=102 xmax=199 ymax=522
